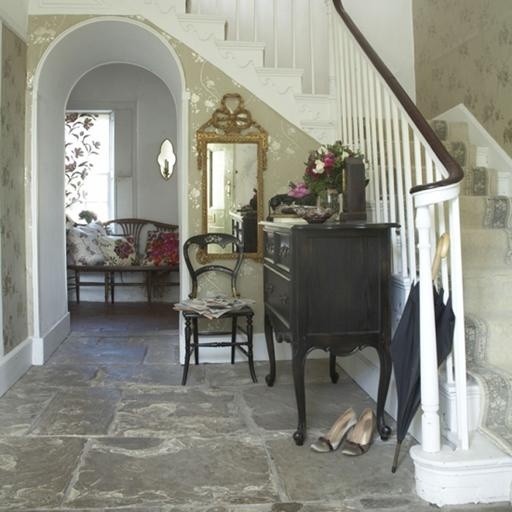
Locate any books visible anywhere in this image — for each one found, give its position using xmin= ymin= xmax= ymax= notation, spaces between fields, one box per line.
xmin=266 ymin=213 xmax=307 ymax=222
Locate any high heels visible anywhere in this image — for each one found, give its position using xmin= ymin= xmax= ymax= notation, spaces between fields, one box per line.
xmin=310 ymin=407 xmax=357 ymax=453
xmin=340 ymin=408 xmax=377 ymax=456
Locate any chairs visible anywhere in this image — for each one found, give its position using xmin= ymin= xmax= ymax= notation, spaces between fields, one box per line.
xmin=182 ymin=233 xmax=258 ymax=386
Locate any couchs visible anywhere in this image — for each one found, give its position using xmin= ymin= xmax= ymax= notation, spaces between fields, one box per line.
xmin=67 ymin=218 xmax=179 ymax=313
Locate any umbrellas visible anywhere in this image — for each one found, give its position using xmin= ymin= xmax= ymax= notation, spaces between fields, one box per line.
xmin=389 ymin=232 xmax=456 ymax=473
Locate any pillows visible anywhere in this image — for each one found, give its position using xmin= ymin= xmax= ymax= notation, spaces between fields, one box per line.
xmin=140 ymin=230 xmax=179 ymax=268
xmin=67 ymin=221 xmax=109 ymax=266
xmin=96 ymin=233 xmax=140 ymax=267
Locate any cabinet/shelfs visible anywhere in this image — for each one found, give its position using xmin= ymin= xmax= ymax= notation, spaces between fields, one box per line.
xmin=258 ymin=219 xmax=401 ymax=446
xmin=229 ymin=208 xmax=257 ymax=253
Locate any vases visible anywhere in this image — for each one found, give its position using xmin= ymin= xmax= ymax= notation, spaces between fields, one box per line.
xmin=296 ymin=207 xmax=306 ymax=217
xmin=319 ymin=191 xmax=340 ymax=224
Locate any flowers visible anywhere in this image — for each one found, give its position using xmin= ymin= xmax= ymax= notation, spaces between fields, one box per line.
xmin=287 ymin=181 xmax=312 ymax=207
xmin=79 ymin=210 xmax=97 ymax=223
xmin=302 ymin=140 xmax=369 ymax=196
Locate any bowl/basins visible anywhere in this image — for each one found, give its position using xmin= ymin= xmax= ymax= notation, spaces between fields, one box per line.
xmin=293 ymin=207 xmax=336 ymax=224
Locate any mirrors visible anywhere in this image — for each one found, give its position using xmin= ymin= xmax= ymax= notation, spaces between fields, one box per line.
xmin=157 ymin=138 xmax=176 ymax=181
xmin=196 ymin=92 xmax=268 ymax=265
